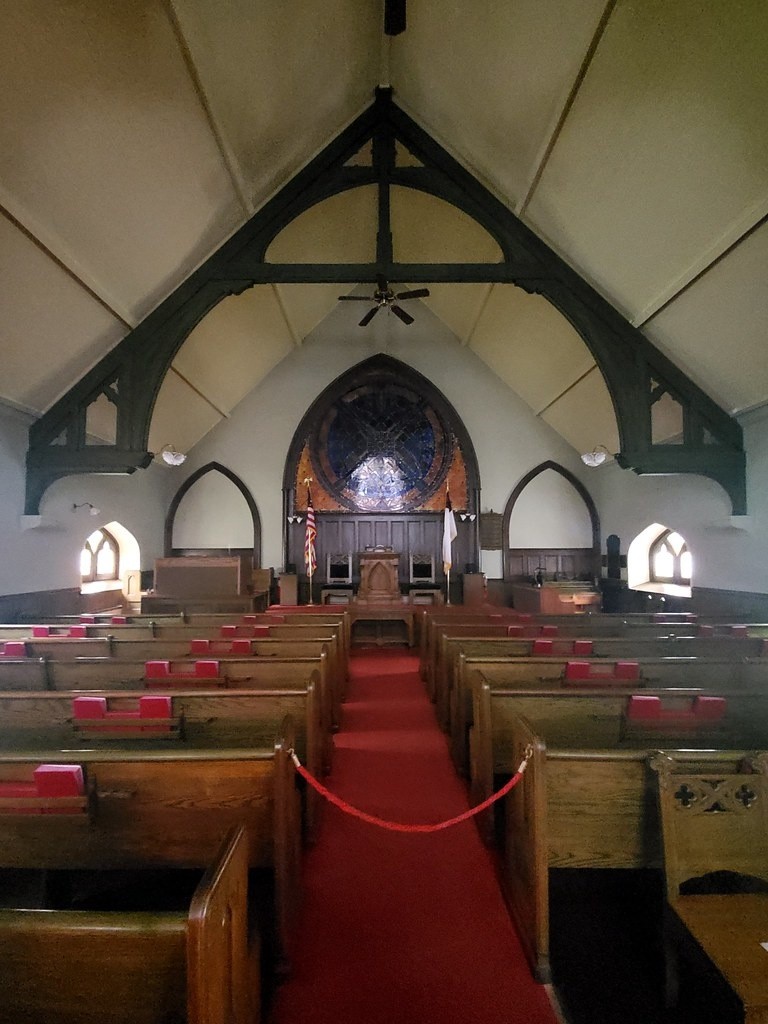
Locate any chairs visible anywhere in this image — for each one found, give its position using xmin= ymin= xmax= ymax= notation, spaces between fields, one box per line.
xmin=408 ymin=554 xmax=441 ymax=606
xmin=321 ymin=554 xmax=353 ymax=604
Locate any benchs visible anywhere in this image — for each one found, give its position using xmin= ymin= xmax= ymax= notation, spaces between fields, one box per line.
xmin=1 ymin=611 xmax=768 ymax=1024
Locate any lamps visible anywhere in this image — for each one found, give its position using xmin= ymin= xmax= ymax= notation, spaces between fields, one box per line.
xmin=581 ymin=444 xmax=616 ymax=468
xmin=149 ymin=443 xmax=187 ymax=466
xmin=74 ymin=503 xmax=100 ymax=516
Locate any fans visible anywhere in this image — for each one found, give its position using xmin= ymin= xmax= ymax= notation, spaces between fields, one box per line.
xmin=338 ymin=284 xmax=429 ymax=327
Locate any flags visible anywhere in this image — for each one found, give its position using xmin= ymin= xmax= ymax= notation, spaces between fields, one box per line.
xmin=443 ymin=501 xmax=458 ymax=575
xmin=304 ymin=487 xmax=318 ymax=577
